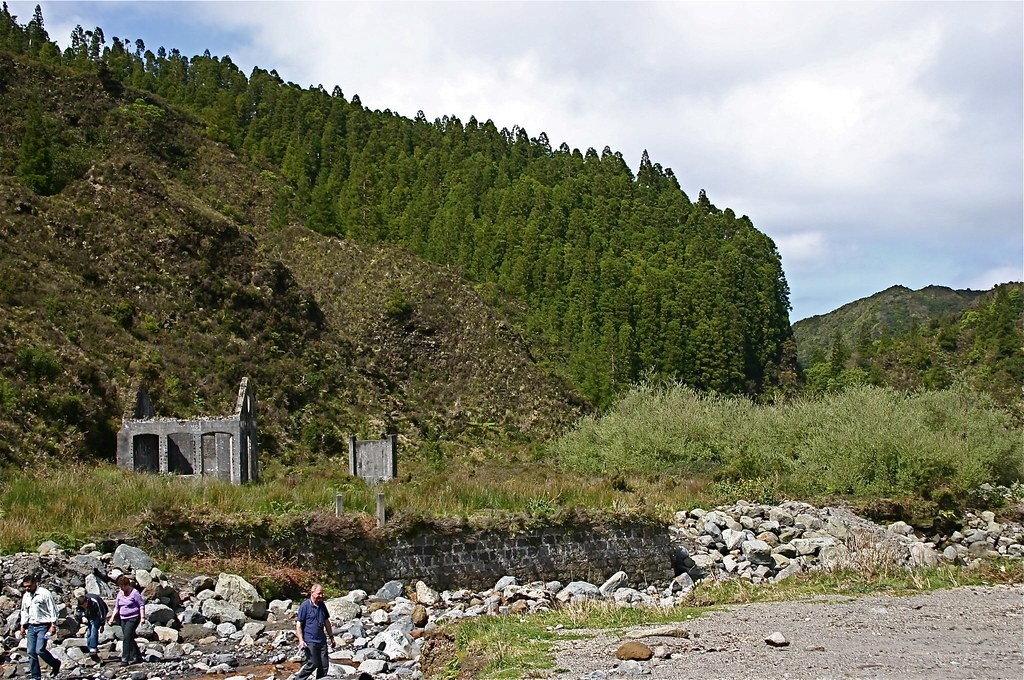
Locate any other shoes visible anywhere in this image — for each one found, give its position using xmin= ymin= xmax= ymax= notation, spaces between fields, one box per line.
xmin=50 ymin=660 xmax=61 ymax=678
xmin=130 ymin=658 xmax=143 ymax=665
xmin=90 ymin=651 xmax=98 ymax=658
xmin=29 ymin=676 xmax=41 ymax=680
xmin=120 ymin=661 xmax=129 ymax=667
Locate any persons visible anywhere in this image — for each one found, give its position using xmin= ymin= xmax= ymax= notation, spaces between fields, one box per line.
xmin=74 ymin=593 xmax=109 ymax=655
xmin=107 ymin=576 xmax=146 ymax=666
xmin=19 ymin=575 xmax=61 ymax=680
xmin=292 ymin=583 xmax=336 ymax=680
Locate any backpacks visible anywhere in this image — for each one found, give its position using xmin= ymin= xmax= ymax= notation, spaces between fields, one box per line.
xmin=77 ymin=594 xmax=109 ymax=626
